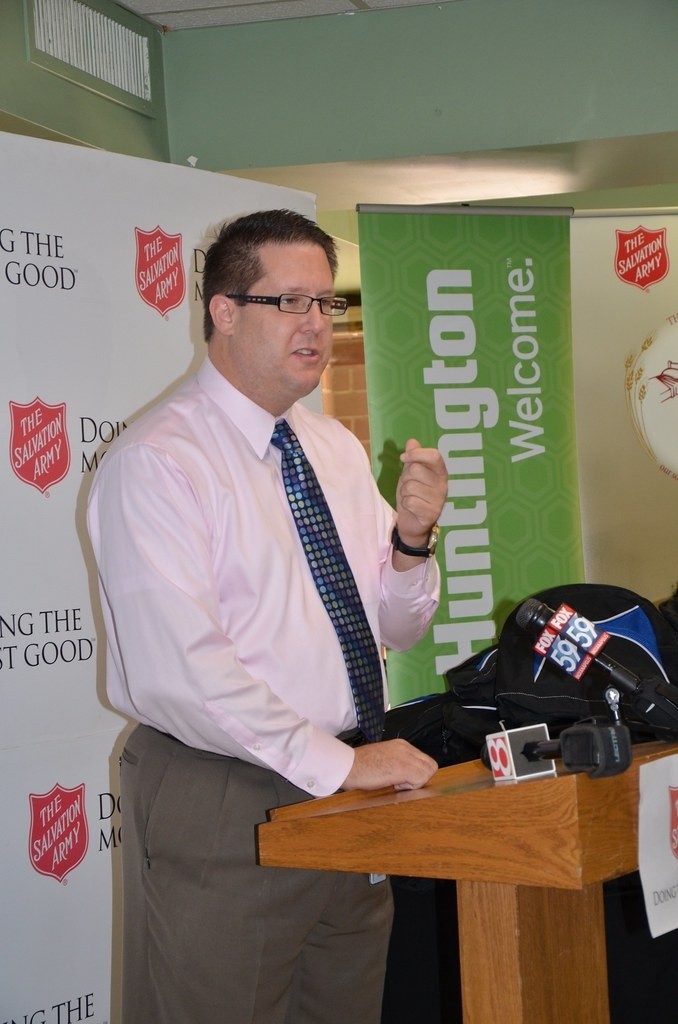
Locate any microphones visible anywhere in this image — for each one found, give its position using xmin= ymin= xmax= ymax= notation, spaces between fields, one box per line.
xmin=480 ymin=723 xmax=632 ymax=783
xmin=515 ymin=599 xmax=678 ymax=729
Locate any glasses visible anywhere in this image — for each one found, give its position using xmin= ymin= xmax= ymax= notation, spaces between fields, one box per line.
xmin=224 ymin=293 xmax=348 ymax=316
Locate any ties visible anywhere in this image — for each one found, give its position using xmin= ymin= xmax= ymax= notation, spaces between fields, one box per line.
xmin=270 ymin=418 xmax=385 ymax=743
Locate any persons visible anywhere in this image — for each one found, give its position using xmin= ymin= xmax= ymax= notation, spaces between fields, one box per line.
xmin=87 ymin=208 xmax=450 ymax=1024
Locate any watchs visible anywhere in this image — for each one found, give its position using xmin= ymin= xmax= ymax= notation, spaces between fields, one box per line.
xmin=391 ymin=523 xmax=440 ymax=558
